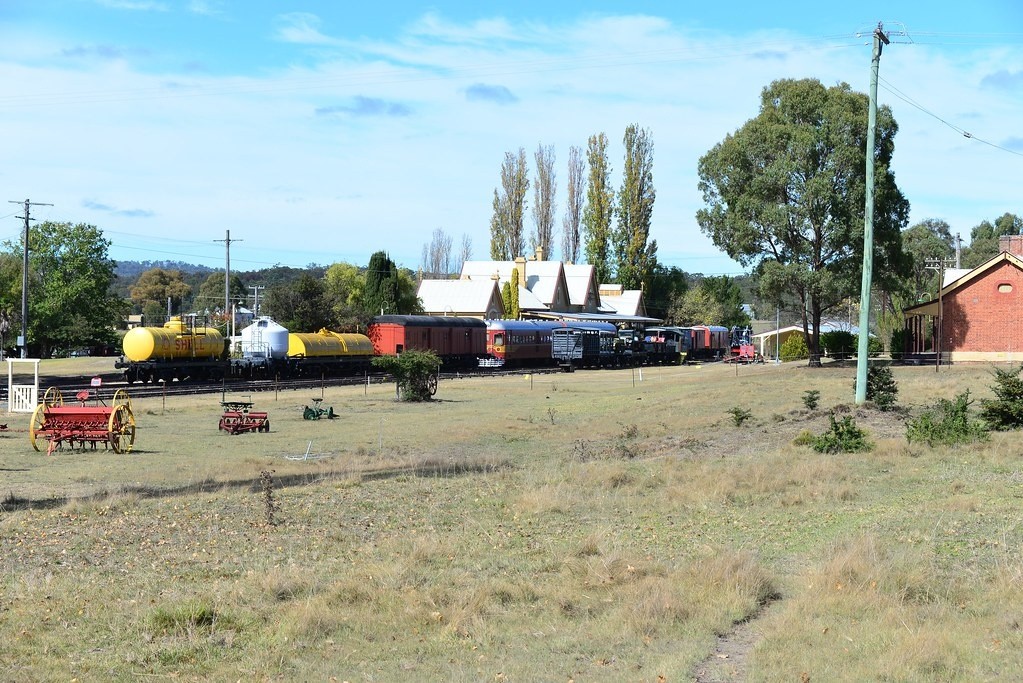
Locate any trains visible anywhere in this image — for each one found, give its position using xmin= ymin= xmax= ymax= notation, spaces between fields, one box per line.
xmin=113 ymin=317 xmax=732 ymax=385
